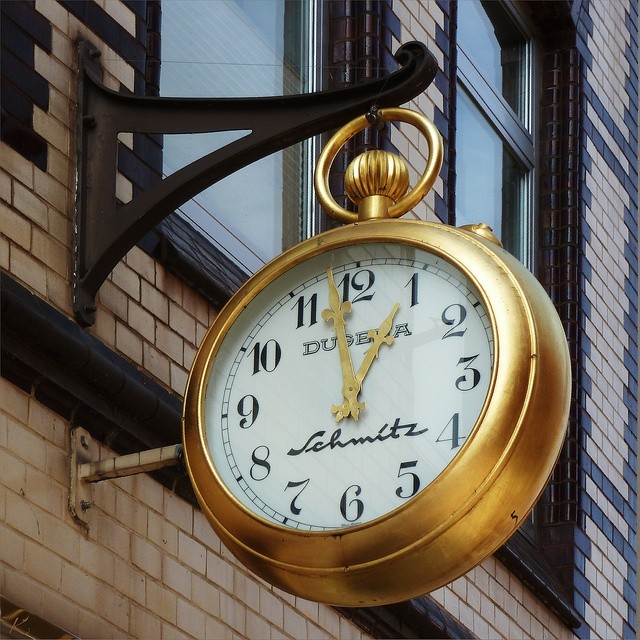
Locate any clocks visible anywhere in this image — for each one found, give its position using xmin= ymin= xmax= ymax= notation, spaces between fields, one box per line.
xmin=180 ymin=221 xmax=571 ymax=605
